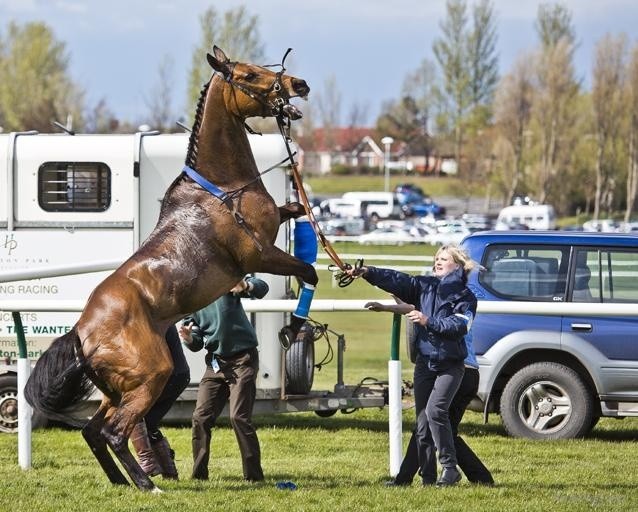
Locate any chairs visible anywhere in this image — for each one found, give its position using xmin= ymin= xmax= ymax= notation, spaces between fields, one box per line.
xmin=574 ymin=266 xmax=592 ymax=302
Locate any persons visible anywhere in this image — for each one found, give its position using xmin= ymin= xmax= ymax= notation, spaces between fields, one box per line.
xmin=346 ymin=247 xmax=481 ymax=487
xmin=510 ymin=221 xmax=530 ymax=258
xmin=512 ymin=194 xmax=539 ymax=206
xmin=177 ymin=273 xmax=269 ymax=482
xmin=365 ymin=267 xmax=496 ymax=487
xmin=496 ymin=217 xmax=510 ymax=230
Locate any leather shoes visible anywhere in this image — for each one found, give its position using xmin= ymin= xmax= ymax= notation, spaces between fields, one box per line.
xmin=436 ymin=465 xmax=462 ymax=487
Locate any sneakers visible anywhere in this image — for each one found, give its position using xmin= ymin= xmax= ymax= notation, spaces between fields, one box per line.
xmin=386 ymin=481 xmax=396 ymax=486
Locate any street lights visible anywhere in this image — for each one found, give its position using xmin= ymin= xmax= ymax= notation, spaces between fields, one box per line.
xmin=382 ymin=137 xmax=393 ymax=191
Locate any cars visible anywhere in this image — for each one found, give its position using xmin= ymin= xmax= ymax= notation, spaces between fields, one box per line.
xmin=312 ymin=183 xmax=445 ymax=220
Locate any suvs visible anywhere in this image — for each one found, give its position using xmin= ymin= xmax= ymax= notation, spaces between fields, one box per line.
xmin=455 ymin=230 xmax=638 ymax=442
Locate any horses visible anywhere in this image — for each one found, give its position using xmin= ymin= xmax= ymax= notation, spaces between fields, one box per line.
xmin=22 ymin=43 xmax=321 ymax=498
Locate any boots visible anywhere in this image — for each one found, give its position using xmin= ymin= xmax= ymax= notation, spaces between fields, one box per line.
xmin=152 ymin=437 xmax=179 ymax=480
xmin=130 ymin=422 xmax=162 ymax=476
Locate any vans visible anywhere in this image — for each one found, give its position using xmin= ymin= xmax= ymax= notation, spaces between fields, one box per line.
xmin=494 ymin=205 xmax=556 ymax=231
